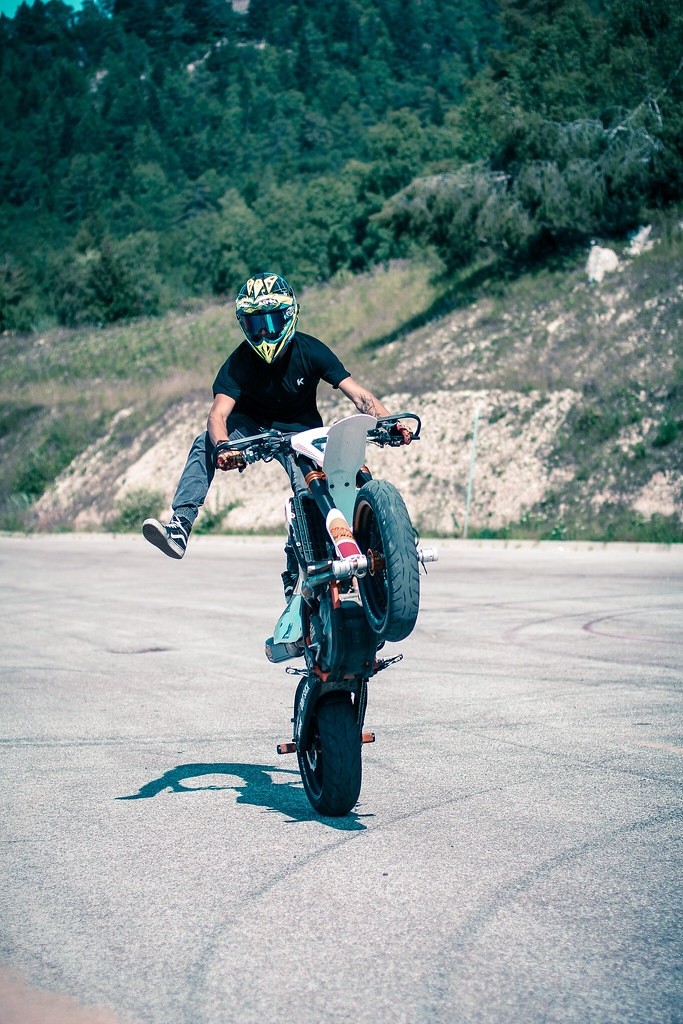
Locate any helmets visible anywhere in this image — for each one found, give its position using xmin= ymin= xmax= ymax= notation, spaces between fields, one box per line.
xmin=235 ymin=272 xmax=299 ymax=364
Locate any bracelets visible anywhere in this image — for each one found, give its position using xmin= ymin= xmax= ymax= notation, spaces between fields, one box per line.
xmin=216 ymin=440 xmax=230 ymax=447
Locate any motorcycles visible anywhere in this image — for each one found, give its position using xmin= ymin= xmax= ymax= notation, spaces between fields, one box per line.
xmin=211 ymin=412 xmax=438 ymax=816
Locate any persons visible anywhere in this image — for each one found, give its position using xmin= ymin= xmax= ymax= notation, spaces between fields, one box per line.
xmin=142 ymin=272 xmax=413 ymax=559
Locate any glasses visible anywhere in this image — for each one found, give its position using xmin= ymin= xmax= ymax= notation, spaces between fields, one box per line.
xmin=240 ymin=308 xmax=286 ymax=335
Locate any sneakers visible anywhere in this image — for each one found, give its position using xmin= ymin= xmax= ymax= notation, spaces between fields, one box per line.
xmin=141 ymin=515 xmax=192 ymax=558
